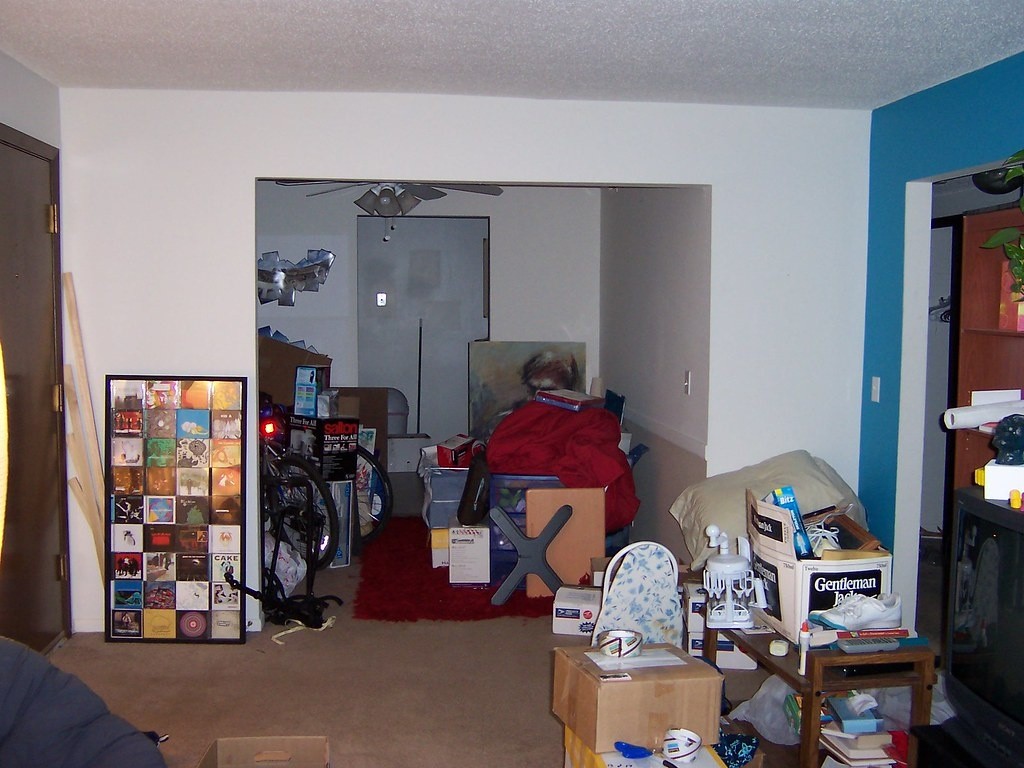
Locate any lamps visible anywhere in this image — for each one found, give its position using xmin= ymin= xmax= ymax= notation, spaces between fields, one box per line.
xmin=353 ymin=183 xmax=421 ymax=218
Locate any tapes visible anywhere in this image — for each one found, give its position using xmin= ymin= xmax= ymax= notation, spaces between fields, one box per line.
xmin=661 ymin=727 xmax=702 ymax=763
xmin=597 ymin=629 xmax=644 ymax=657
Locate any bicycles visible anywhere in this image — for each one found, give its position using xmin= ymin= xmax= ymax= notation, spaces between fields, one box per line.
xmin=259 ymin=392 xmax=393 ymax=571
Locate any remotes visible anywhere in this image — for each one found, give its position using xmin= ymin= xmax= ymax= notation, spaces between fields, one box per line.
xmin=837 ymin=637 xmax=899 ymax=654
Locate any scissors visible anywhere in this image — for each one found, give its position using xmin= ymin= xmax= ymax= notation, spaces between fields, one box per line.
xmin=614 ymin=741 xmax=664 ymax=758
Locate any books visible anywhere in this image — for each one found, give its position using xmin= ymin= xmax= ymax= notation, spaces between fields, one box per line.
xmin=820 ymin=727 xmax=896 ymax=765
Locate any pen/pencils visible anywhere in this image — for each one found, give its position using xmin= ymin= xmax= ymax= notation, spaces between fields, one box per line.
xmin=663 ymin=759 xmax=678 ymax=768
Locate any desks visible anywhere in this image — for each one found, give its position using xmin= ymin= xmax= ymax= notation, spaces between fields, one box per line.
xmin=701 ymin=592 xmax=938 ymax=768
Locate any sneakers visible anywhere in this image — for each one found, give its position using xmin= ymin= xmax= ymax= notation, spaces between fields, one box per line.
xmin=808 ymin=592 xmax=903 ymax=631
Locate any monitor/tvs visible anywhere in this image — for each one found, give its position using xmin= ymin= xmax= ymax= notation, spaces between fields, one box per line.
xmin=944 ymin=486 xmax=1024 ymax=768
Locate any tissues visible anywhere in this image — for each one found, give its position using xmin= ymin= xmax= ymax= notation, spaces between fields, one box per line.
xmin=827 ymin=692 xmax=884 ymax=735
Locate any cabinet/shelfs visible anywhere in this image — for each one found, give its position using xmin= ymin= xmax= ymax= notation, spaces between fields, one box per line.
xmin=942 ymin=200 xmax=1024 ymax=726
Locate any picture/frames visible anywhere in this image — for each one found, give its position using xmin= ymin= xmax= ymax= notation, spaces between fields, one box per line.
xmin=825 ymin=511 xmax=882 ymax=549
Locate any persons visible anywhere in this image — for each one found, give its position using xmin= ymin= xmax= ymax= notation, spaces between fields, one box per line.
xmin=1 ymin=634 xmax=165 ymax=768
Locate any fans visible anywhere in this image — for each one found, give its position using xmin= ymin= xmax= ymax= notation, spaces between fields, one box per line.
xmin=274 ymin=179 xmax=504 ymax=201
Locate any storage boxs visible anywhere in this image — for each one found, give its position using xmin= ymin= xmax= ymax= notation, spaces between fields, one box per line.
xmin=564 ymin=725 xmax=728 ymax=768
xmin=682 ymin=583 xmax=724 ymax=633
xmin=489 ymin=474 xmax=561 ymax=588
xmin=998 ymin=261 xmax=1024 ymax=332
xmin=196 ymin=735 xmax=331 ymax=768
xmin=436 ymin=433 xmax=476 ymax=471
xmin=984 ymin=458 xmax=1024 ymax=501
xmin=590 ymin=557 xmax=624 ymax=584
xmin=287 ymin=413 xmax=359 ymax=483
xmin=448 ymin=515 xmax=491 ymax=583
xmin=294 ymin=367 xmax=325 ymax=417
xmin=552 ymin=584 xmax=603 ymax=635
xmin=550 ymin=642 xmax=726 ymax=754
xmin=761 ymin=486 xmax=814 ymax=560
xmin=317 ymin=395 xmax=339 ymax=417
xmin=745 ymin=487 xmax=893 ymax=645
xmin=689 ymin=633 xmax=758 ymax=670
xmin=827 ymin=697 xmax=884 ymax=734
xmin=308 ymin=480 xmax=353 ymax=569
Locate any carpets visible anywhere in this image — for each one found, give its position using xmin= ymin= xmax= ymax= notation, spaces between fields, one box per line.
xmin=349 ymin=513 xmax=556 ymax=624
